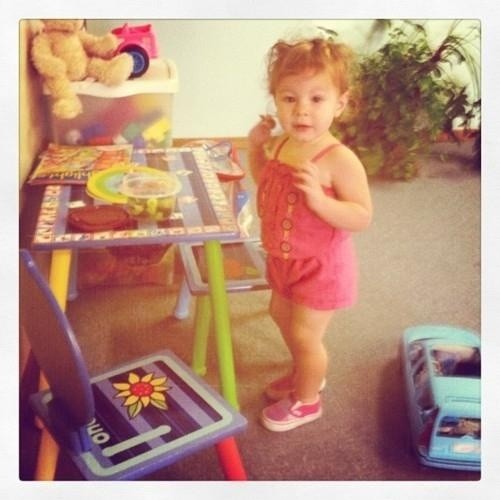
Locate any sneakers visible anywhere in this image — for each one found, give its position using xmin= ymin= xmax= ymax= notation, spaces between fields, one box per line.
xmin=260 ymin=392 xmax=323 ymax=432
xmin=266 ymin=364 xmax=326 ymax=400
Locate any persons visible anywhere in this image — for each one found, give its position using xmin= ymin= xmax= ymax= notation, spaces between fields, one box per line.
xmin=247 ymin=37 xmax=373 ymax=433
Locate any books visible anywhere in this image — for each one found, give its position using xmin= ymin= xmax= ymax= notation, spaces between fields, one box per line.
xmin=26 ymin=143 xmax=134 ymax=183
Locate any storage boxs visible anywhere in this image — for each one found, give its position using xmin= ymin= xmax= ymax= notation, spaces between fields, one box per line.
xmin=79 ymin=247 xmax=174 ymax=288
xmin=20 ymin=146 xmax=239 ymax=430
xmin=41 ymin=57 xmax=179 ymax=151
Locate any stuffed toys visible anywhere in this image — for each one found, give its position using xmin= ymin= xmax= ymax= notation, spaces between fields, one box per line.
xmin=29 ymin=20 xmax=134 ymax=119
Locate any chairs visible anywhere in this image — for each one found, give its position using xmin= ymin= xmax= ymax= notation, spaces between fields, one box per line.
xmin=20 ymin=247 xmax=248 ymax=480
xmin=173 ymin=237 xmax=272 ymax=377
